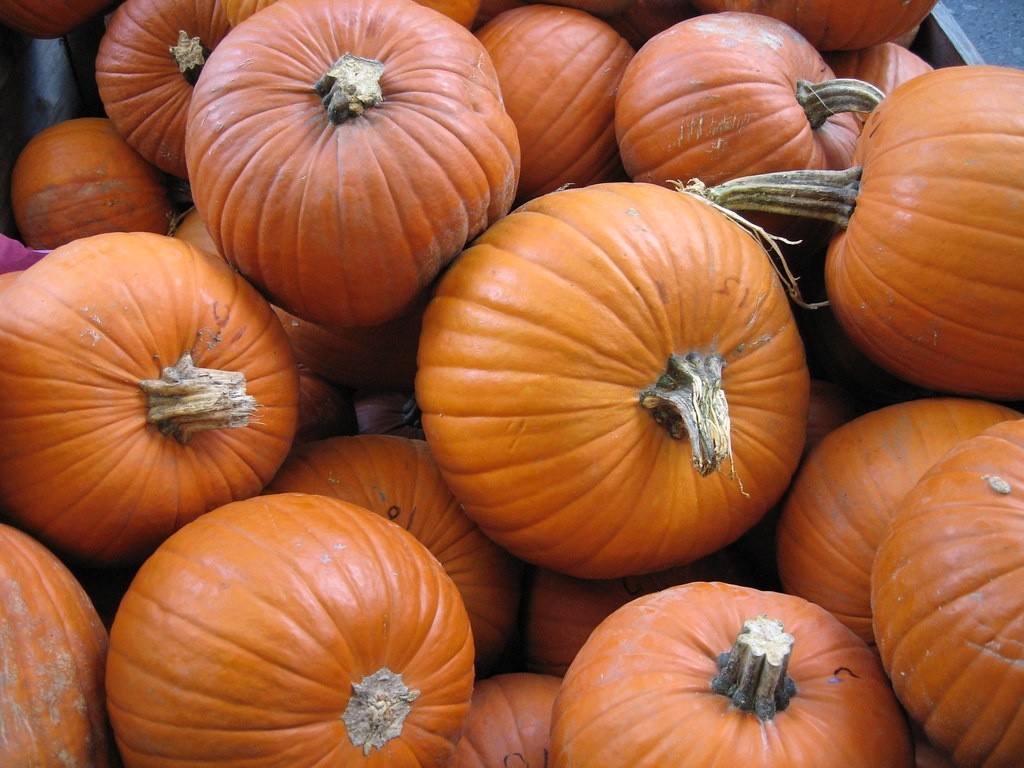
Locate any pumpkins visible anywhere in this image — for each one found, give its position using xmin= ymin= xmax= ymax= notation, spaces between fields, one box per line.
xmin=0 ymin=1 xmax=1024 ymax=768
